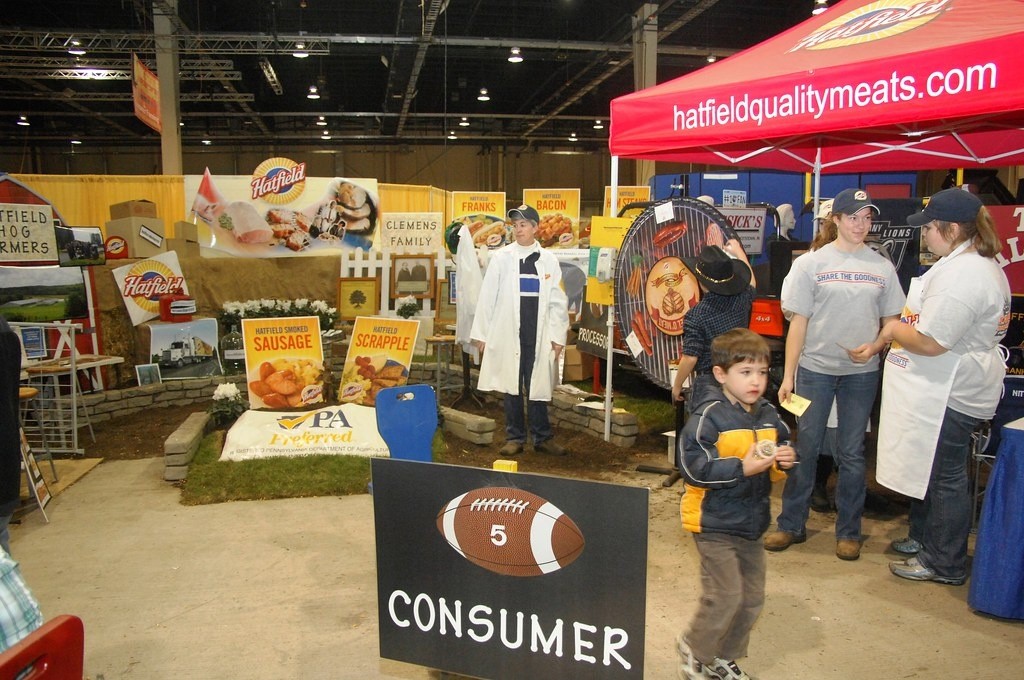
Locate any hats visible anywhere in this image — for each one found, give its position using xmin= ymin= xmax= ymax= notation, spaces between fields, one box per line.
xmin=832 ymin=190 xmax=881 ymax=219
xmin=907 ymin=190 xmax=983 ymax=228
xmin=680 ymin=246 xmax=752 ymax=296
xmin=507 ymin=203 xmax=539 ymax=225
xmin=811 ymin=197 xmax=837 ymax=219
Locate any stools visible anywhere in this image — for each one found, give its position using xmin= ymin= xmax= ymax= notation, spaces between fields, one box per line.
xmin=19 ymin=387 xmax=59 ymax=482
xmin=421 ymin=336 xmax=464 ymax=407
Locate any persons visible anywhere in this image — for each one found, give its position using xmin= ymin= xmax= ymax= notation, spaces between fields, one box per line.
xmin=763 ymin=188 xmax=906 ymax=560
xmin=765 ymin=203 xmax=796 ymax=258
xmin=471 ymin=204 xmax=569 ymax=455
xmin=812 ymin=199 xmax=872 ymax=511
xmin=674 ymin=327 xmax=799 ymax=680
xmin=674 ymin=239 xmax=755 ymax=415
xmin=885 ymin=187 xmax=1011 ymax=586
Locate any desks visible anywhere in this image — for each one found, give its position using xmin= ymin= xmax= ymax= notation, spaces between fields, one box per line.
xmin=27 ymin=354 xmax=124 ymax=391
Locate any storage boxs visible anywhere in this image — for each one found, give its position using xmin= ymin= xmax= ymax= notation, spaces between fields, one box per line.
xmin=750 ymin=299 xmax=784 ymax=338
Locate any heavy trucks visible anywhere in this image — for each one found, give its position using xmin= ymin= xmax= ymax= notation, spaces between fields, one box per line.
xmin=162 ymin=335 xmax=213 ymax=369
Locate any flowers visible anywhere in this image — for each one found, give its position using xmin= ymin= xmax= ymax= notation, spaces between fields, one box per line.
xmin=222 ymin=297 xmax=334 ymax=320
xmin=215 ymin=382 xmax=240 ymax=402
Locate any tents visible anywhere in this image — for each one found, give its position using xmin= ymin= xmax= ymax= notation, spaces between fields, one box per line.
xmin=604 ymin=0 xmax=1024 ymax=444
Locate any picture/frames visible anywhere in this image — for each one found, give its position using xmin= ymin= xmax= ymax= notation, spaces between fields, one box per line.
xmin=135 ymin=363 xmax=162 ymax=386
xmin=435 ymin=279 xmax=456 ymax=325
xmin=335 ymin=277 xmax=380 ymax=322
xmin=390 ymin=254 xmax=436 ymax=299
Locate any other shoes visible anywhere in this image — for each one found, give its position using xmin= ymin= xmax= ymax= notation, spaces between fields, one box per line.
xmin=501 ymin=442 xmax=523 ymax=455
xmin=534 ymin=439 xmax=565 ymax=454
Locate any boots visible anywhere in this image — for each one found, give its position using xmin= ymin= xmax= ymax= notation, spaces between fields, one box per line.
xmin=811 ymin=455 xmax=833 ymax=511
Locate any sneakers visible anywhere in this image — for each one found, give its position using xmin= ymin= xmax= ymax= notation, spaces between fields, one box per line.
xmin=763 ymin=529 xmax=803 ymax=551
xmin=675 ymin=632 xmax=711 ymax=680
xmin=892 ymin=534 xmax=927 ymax=554
xmin=703 ymin=653 xmax=750 ymax=680
xmin=890 ymin=556 xmax=966 ymax=585
xmin=837 ymin=536 xmax=859 ymax=561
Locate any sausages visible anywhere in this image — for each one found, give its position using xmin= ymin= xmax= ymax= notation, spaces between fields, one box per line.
xmin=632 ymin=312 xmax=653 ymax=357
xmin=652 ymin=221 xmax=688 ymax=248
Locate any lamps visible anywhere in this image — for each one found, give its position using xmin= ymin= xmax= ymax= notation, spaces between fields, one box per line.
xmin=448 ymin=130 xmax=457 ymax=139
xmin=17 ymin=115 xmax=30 ymax=125
xmin=570 ymin=131 xmax=580 ymax=142
xmin=321 ymin=130 xmax=332 ymax=139
xmin=459 ymin=118 xmax=470 ymax=126
xmin=68 ymin=37 xmax=86 ymax=55
xmin=307 ymin=82 xmax=320 ymax=99
xmin=593 ymin=118 xmax=603 ymax=129
xmin=477 ymin=86 xmax=490 ymax=101
xmin=811 ymin=0 xmax=829 ymax=15
xmin=508 ymin=47 xmax=524 ymax=62
xmin=293 ymin=35 xmax=308 ymax=57
xmin=317 ymin=114 xmax=327 ymax=125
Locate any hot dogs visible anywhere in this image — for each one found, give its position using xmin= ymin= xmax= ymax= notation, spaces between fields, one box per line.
xmin=465 ymin=220 xmax=506 ymax=247
